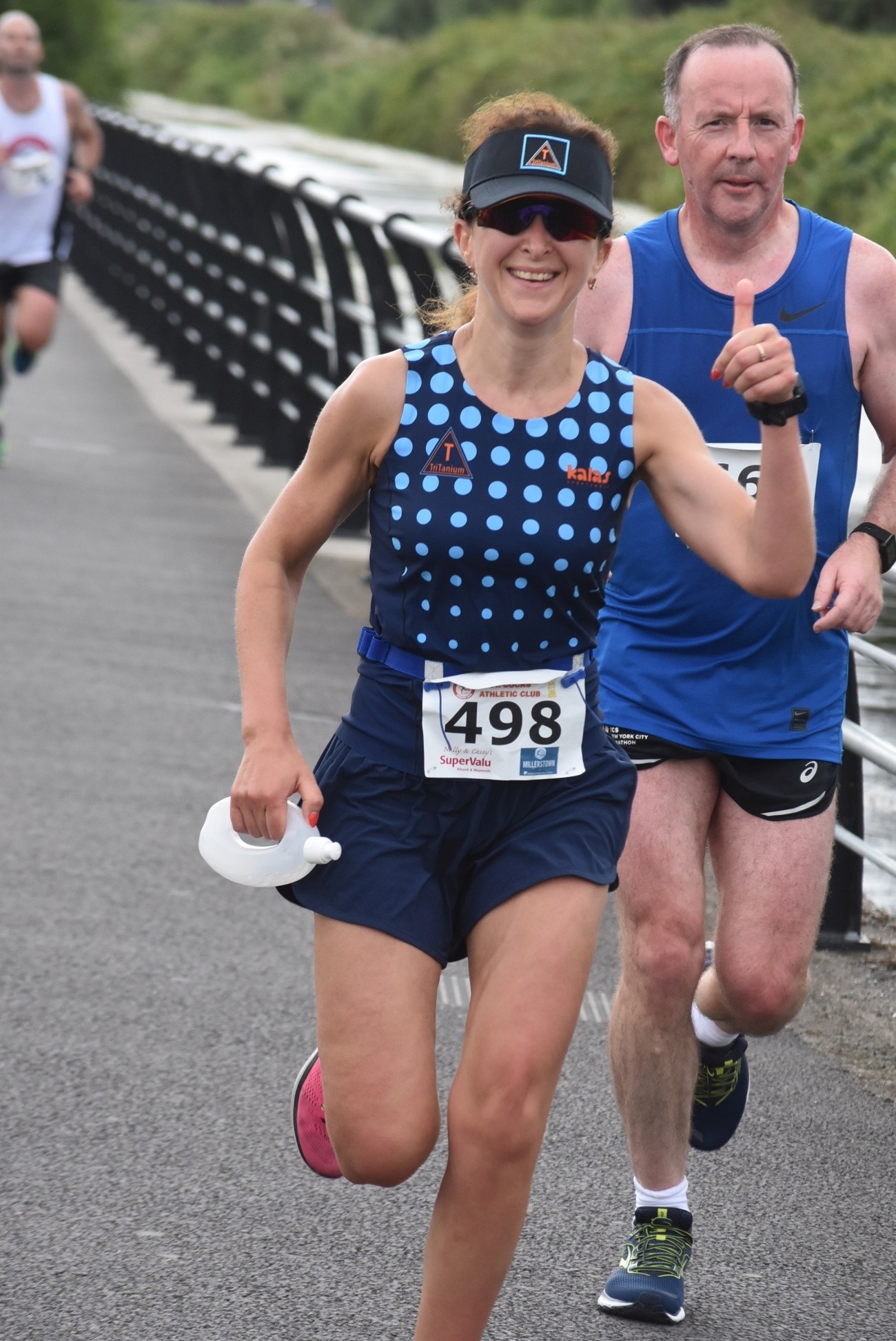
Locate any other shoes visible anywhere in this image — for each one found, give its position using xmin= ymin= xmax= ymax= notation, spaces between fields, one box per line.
xmin=12 ymin=346 xmax=34 ymax=374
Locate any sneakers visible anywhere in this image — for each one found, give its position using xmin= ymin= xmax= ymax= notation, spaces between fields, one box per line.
xmin=597 ymin=1206 xmax=693 ymax=1324
xmin=684 ymin=940 xmax=751 ymax=1152
xmin=291 ymin=1049 xmax=343 ymax=1179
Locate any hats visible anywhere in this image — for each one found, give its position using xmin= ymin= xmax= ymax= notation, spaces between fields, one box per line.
xmin=461 ymin=123 xmax=612 ymax=231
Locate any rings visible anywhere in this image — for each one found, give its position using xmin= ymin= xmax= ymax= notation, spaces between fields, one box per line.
xmin=754 ymin=343 xmax=767 ymax=361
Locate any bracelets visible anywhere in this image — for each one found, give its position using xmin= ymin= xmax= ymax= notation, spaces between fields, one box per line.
xmin=745 ymin=371 xmax=809 ymax=428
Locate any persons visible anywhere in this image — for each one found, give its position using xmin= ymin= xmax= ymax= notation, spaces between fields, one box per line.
xmin=571 ymin=21 xmax=896 ymax=1320
xmin=230 ymin=91 xmax=815 ymax=1341
xmin=0 ymin=9 xmax=102 ymax=387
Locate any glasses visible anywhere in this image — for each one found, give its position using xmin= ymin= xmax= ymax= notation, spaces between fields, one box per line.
xmin=476 ymin=193 xmax=606 ymax=243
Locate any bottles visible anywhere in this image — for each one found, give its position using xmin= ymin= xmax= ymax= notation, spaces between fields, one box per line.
xmin=197 ymin=796 xmax=343 ymax=888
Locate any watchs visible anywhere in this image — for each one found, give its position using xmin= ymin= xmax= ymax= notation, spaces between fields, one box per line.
xmin=848 ymin=521 xmax=896 ymax=574
